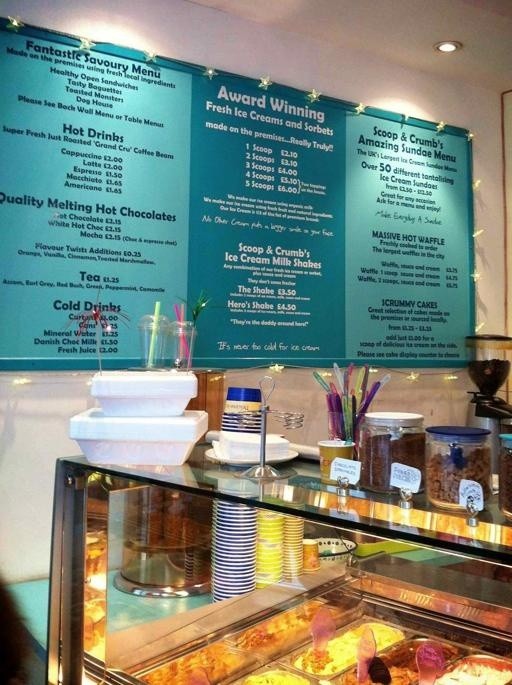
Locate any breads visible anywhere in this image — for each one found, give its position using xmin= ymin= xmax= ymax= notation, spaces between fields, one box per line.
xmin=139 ymin=644 xmax=249 ymax=685
xmin=237 ymin=602 xmax=340 ymax=656
xmin=82 ymin=539 xmax=108 ymax=653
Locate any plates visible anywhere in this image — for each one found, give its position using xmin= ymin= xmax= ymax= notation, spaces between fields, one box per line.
xmin=203 ymin=444 xmax=300 ymax=466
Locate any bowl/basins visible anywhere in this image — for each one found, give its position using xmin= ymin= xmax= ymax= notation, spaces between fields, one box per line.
xmin=313 ymin=537 xmax=357 ymax=566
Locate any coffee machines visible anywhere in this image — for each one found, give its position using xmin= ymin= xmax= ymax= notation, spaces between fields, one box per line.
xmin=463 ymin=333 xmax=512 ymax=475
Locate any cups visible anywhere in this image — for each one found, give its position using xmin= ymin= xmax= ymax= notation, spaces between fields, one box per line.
xmin=137 ymin=315 xmax=168 ymax=369
xmin=317 ymin=412 xmax=362 ymax=486
xmin=169 ymin=321 xmax=197 ymax=374
xmin=301 ymin=538 xmax=321 ymax=571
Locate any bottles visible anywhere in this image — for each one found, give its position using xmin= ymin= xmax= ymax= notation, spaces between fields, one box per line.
xmin=498 ymin=433 xmax=512 ymax=523
xmin=426 ymin=426 xmax=492 ymax=513
xmin=361 ymin=412 xmax=426 ymax=495
